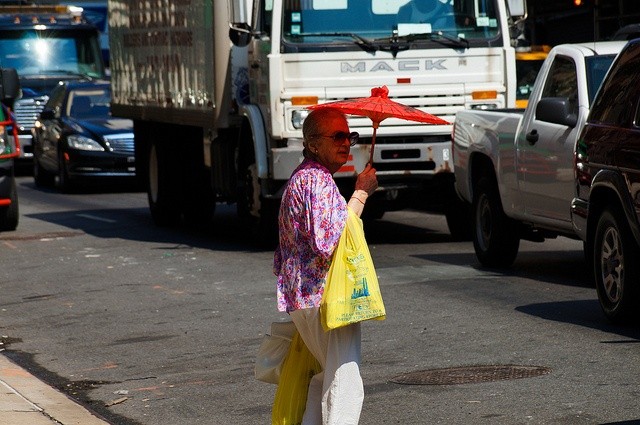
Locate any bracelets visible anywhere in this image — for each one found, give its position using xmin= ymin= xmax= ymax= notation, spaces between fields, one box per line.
xmin=351 ymin=196 xmax=364 ymax=205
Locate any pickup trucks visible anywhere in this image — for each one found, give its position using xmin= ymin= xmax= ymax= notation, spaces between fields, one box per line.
xmin=452 ymin=40 xmax=628 ymax=269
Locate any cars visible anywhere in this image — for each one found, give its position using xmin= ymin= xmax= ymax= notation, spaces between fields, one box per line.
xmin=30 ymin=79 xmax=135 ymax=194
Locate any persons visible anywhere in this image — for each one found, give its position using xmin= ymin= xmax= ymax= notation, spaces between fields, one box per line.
xmin=272 ymin=108 xmax=379 ymax=424
xmin=395 ymin=0 xmax=452 ymax=28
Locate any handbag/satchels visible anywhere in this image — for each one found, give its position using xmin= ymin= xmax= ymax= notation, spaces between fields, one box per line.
xmin=255 ymin=322 xmax=297 ymax=384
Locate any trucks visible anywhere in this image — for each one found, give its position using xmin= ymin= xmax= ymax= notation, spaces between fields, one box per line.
xmin=1 ymin=3 xmax=108 ymax=159
xmin=107 ymin=0 xmax=529 ymax=235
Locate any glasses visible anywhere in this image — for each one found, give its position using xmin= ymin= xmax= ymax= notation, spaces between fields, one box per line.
xmin=308 ymin=131 xmax=359 ymax=146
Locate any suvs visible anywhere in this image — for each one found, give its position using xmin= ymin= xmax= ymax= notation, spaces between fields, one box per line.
xmin=1 ymin=67 xmax=22 ymax=232
xmin=569 ymin=37 xmax=640 ymax=322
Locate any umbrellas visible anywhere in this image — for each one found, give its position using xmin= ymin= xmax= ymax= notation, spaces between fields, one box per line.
xmin=307 ymin=85 xmax=455 ymax=170
